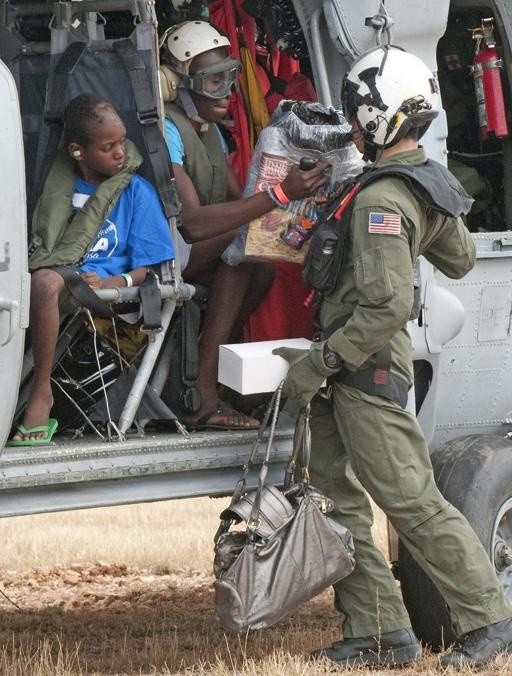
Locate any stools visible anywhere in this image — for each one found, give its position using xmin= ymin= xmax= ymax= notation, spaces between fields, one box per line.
xmin=13 ymin=283 xmax=196 ymax=442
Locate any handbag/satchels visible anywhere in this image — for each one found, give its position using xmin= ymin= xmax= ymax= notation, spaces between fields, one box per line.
xmin=212 ymin=487 xmax=355 ymax=634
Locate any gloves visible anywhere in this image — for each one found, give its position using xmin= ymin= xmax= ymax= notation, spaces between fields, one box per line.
xmin=272 ymin=340 xmax=344 ymax=416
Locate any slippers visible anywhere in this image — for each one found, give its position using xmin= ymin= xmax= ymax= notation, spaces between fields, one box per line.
xmin=9 ymin=418 xmax=59 ymax=447
xmin=186 ymin=402 xmax=261 ymax=429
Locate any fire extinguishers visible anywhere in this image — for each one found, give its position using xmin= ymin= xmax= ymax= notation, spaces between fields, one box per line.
xmin=469 ymin=26 xmax=510 ymax=145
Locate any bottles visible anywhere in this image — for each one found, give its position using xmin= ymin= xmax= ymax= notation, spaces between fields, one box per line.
xmin=321 ymin=239 xmax=337 ymax=258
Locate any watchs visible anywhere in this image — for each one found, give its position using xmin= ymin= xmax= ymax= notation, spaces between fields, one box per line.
xmin=322 ymin=343 xmax=342 ymax=369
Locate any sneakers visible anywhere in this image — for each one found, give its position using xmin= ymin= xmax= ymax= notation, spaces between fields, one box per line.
xmin=436 ymin=619 xmax=510 ymax=669
xmin=307 ymin=628 xmax=420 ymax=669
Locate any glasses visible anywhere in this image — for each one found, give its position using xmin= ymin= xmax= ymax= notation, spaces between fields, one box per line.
xmin=192 ymin=56 xmax=243 ymax=100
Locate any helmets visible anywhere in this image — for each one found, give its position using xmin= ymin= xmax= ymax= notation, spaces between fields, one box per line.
xmin=342 ymin=48 xmax=443 ymax=149
xmin=158 ymin=21 xmax=234 ymax=63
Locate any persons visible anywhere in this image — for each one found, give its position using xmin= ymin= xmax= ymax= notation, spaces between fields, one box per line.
xmin=265 ymin=45 xmax=510 ymax=674
xmin=5 ymin=90 xmax=175 ymax=450
xmin=155 ymin=18 xmax=335 ymax=435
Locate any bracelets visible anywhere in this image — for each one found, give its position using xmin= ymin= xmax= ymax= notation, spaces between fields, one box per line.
xmin=119 ymin=269 xmax=134 ymax=288
xmin=271 ymin=182 xmax=293 ymax=205
xmin=266 ymin=185 xmax=285 ymax=210
